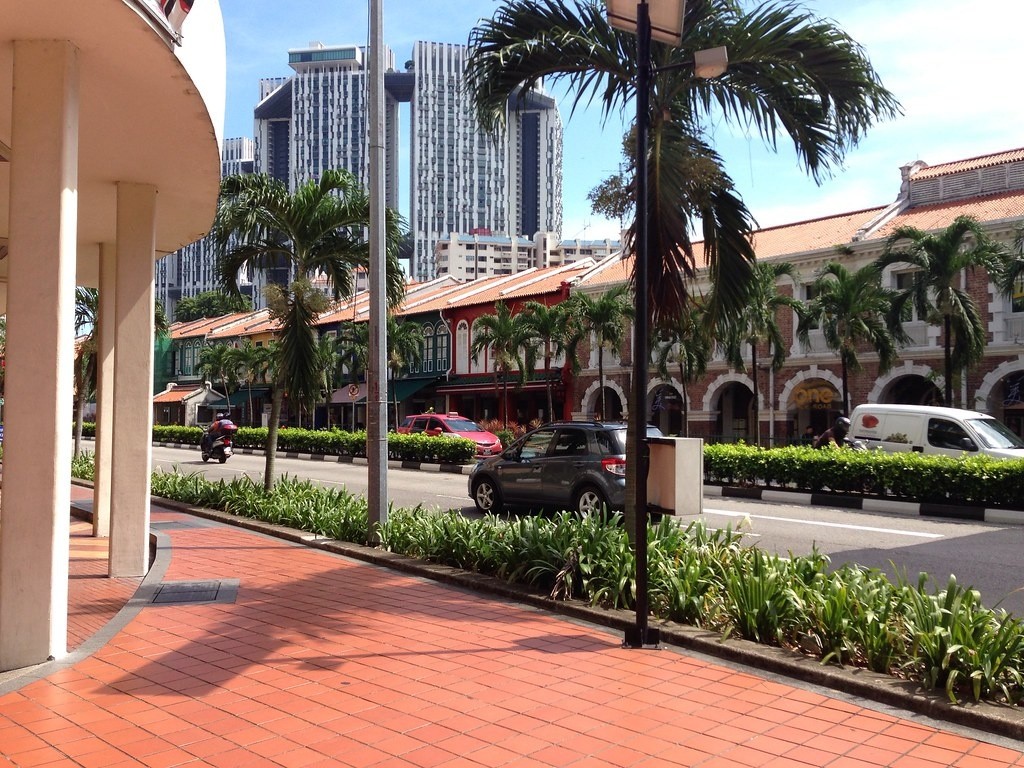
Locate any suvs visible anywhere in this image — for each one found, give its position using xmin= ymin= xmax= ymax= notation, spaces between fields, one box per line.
xmin=468 ymin=420 xmax=666 ymax=528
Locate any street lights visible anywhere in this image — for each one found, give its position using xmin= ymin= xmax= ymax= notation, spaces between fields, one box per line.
xmin=622 ymin=46 xmax=731 ymax=650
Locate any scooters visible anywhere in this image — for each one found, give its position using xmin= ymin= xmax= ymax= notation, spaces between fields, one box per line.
xmin=198 ymin=423 xmax=238 ymax=464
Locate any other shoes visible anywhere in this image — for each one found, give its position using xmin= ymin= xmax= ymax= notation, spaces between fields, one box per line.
xmin=205 ymin=449 xmax=213 ymax=453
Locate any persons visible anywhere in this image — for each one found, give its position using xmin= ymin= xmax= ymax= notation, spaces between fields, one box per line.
xmin=202 ymin=413 xmax=233 ymax=455
xmin=802 ymin=417 xmax=856 ymax=450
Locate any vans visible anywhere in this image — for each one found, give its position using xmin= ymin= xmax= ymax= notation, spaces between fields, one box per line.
xmin=846 ymin=404 xmax=1024 ymax=460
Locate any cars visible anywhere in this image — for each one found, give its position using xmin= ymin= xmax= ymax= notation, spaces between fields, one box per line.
xmin=397 ymin=411 xmax=503 ymax=460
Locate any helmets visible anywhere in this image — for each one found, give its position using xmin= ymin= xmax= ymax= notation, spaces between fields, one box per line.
xmin=224 ymin=412 xmax=231 ymax=419
xmin=216 ymin=412 xmax=224 ymax=420
xmin=836 ymin=417 xmax=852 ymax=436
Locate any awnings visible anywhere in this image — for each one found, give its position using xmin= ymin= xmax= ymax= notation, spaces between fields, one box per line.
xmin=316 ymin=383 xmax=367 ymax=403
xmin=208 ymin=389 xmax=268 ymax=409
xmin=356 ymin=378 xmax=435 ymax=405
